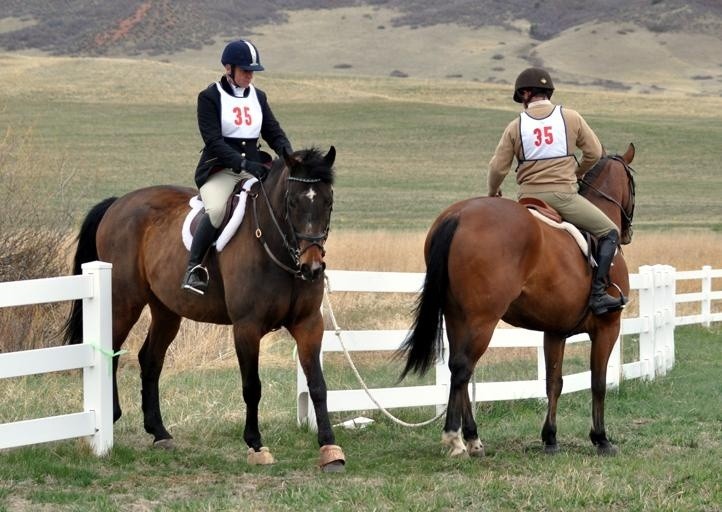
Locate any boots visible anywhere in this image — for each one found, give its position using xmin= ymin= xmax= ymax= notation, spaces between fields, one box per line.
xmin=183 ymin=213 xmax=220 ymax=291
xmin=589 ymin=229 xmax=629 ymax=315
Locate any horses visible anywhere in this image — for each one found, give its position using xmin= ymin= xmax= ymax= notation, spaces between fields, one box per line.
xmin=394 ymin=141 xmax=636 ymax=463
xmin=55 ymin=146 xmax=347 ymax=474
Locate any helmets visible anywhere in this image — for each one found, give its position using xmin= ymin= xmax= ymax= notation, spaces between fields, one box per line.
xmin=221 ymin=40 xmax=264 ymax=71
xmin=513 ymin=67 xmax=555 ymax=103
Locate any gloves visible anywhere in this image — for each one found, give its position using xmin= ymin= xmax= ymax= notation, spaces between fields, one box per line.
xmin=241 ymin=159 xmax=268 ymax=183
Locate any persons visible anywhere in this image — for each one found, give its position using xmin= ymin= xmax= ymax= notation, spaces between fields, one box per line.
xmin=182 ymin=40 xmax=294 ymax=295
xmin=487 ymin=68 xmax=629 ymax=315
